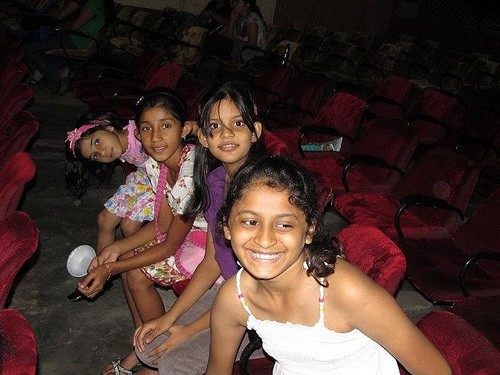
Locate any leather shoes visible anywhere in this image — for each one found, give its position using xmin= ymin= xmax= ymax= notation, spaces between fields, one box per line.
xmin=67 ymin=278 xmax=113 ymax=303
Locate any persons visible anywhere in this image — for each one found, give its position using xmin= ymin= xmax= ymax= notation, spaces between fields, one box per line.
xmin=15 ymin=0 xmax=117 ymax=96
xmin=199 ymin=0 xmax=274 ymax=79
xmin=65 ymin=109 xmax=161 ymax=302
xmin=100 ymin=80 xmax=268 ymax=375
xmin=204 ymin=154 xmax=453 ymax=375
xmin=78 ymin=86 xmax=207 ymax=329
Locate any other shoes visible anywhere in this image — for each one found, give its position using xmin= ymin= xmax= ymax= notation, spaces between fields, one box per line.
xmin=25 ymin=76 xmax=44 ymax=86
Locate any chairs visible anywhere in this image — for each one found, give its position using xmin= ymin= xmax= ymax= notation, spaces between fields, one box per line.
xmin=0 ymin=1 xmax=500 ymax=375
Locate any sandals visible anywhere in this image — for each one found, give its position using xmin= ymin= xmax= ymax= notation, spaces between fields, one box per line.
xmin=98 ymin=358 xmax=133 ymax=375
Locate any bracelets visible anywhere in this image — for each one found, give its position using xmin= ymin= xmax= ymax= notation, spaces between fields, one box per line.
xmin=104 ymin=263 xmax=112 ymax=281
